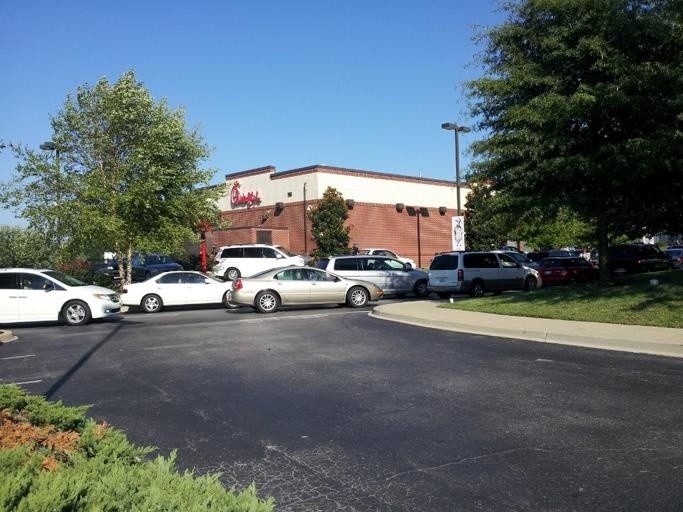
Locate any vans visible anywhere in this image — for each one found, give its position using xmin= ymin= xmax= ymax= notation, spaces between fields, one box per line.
xmin=316 ymin=254 xmax=430 ymax=298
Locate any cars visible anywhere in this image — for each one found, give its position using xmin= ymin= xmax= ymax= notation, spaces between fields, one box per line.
xmin=0 ymin=267 xmax=123 ymax=326
xmin=426 ymin=243 xmax=682 ymax=298
xmin=211 ymin=243 xmax=315 ymax=281
xmin=129 ymin=253 xmax=184 ymax=281
xmin=115 ymin=270 xmax=241 ymax=313
xmin=229 ymin=264 xmax=384 ymax=314
xmin=353 ymin=249 xmax=416 ymax=270
xmin=85 ymin=255 xmax=129 ymax=289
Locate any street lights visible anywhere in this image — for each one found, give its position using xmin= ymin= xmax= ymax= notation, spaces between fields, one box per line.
xmin=40 ymin=141 xmax=73 ymax=225
xmin=441 ymin=122 xmax=472 ymax=216
